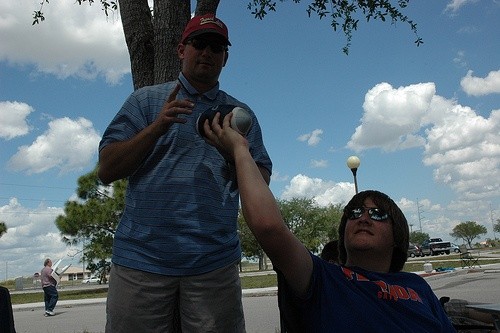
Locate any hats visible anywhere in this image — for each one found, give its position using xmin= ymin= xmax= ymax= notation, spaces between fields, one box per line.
xmin=181 ymin=14 xmax=232 ymax=50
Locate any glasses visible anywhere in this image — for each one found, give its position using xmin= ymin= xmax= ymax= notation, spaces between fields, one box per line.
xmin=183 ymin=37 xmax=228 ymax=53
xmin=344 ymin=205 xmax=393 ymax=223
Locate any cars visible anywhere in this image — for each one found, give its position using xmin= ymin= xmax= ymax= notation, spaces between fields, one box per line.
xmin=407 ymin=237 xmax=460 ymax=258
xmin=81 ymin=276 xmax=101 ymax=283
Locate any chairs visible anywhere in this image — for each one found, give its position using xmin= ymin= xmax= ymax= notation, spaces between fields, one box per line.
xmin=50 ymin=258 xmax=73 ymax=284
xmin=458 ymin=244 xmax=482 ymax=269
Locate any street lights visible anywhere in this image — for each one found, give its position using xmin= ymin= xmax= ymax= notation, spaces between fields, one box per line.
xmin=346 ymin=156 xmax=361 ymax=195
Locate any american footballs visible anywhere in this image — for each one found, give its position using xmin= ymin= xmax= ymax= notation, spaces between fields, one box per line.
xmin=196 ymin=104 xmax=252 ymax=139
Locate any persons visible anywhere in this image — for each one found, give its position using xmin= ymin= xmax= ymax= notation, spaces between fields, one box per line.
xmin=321 ymin=240 xmax=340 ymax=265
xmin=97 ymin=13 xmax=272 ymax=333
xmin=198 ymin=112 xmax=457 ymax=333
xmin=41 ymin=258 xmax=60 ymax=317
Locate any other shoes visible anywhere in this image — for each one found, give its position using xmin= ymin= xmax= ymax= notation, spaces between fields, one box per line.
xmin=44 ymin=310 xmax=55 ymax=316
